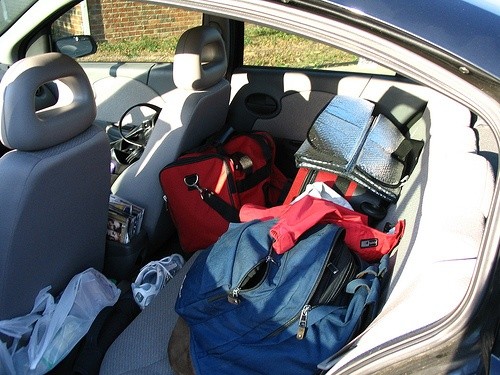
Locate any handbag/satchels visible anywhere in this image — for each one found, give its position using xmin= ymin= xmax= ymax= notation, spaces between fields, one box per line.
xmin=282 ymin=167 xmax=385 ymax=229
xmin=0 ymin=267 xmax=121 ymax=374
xmin=158 ymin=130 xmax=289 ymax=250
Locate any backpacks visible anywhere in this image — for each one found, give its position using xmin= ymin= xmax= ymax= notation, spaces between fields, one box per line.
xmin=174 ymin=216 xmax=388 ymax=375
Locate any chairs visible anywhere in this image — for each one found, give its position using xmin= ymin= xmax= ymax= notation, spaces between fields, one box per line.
xmin=101 ymin=90 xmax=492 ymax=374
xmin=0 ymin=53 xmax=113 ymax=350
xmin=110 ymin=25 xmax=231 ymax=239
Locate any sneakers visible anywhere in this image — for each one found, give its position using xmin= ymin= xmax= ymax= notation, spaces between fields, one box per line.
xmin=130 ymin=255 xmax=185 ymax=308
